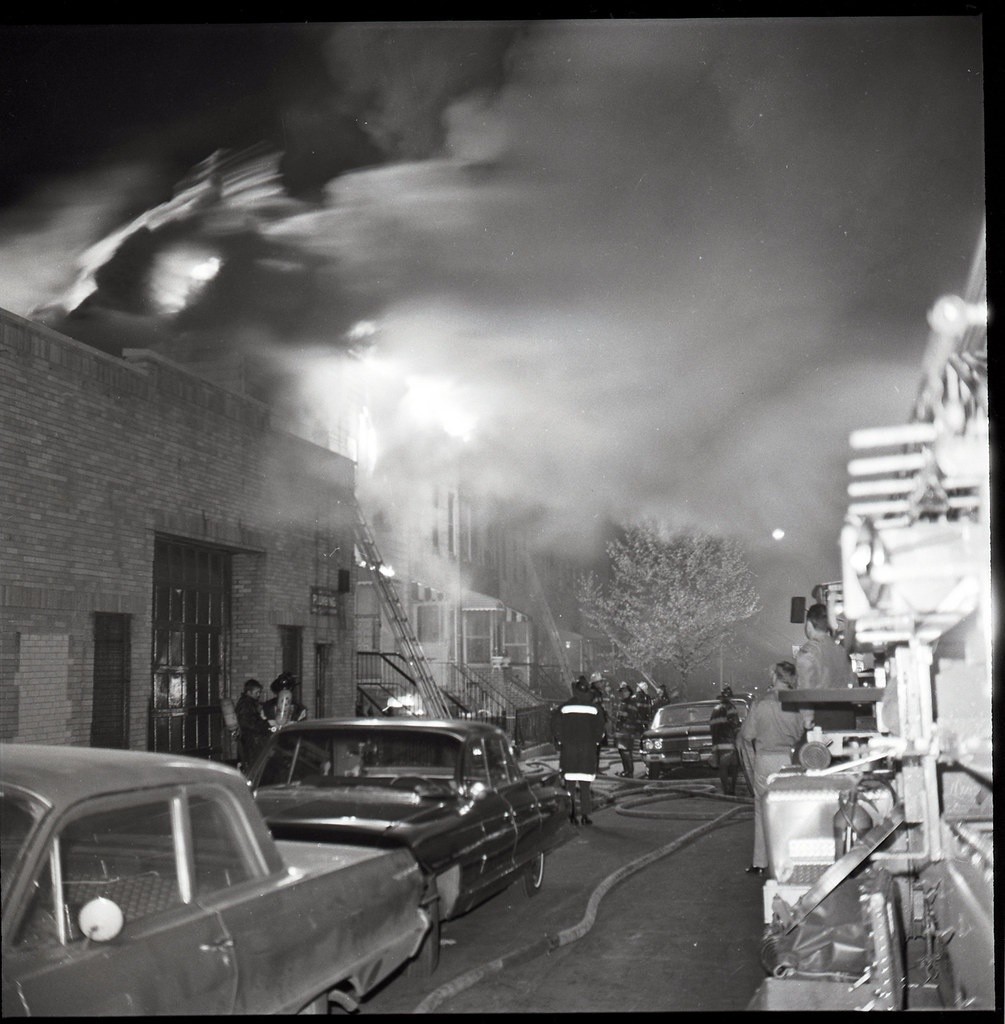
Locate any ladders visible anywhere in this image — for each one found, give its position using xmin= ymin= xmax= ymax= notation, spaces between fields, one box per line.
xmin=523 ymin=552 xmax=575 ymax=696
xmin=338 ymin=485 xmax=452 ymax=720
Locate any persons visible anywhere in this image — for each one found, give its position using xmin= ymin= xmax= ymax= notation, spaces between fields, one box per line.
xmin=710 ymin=687 xmax=743 ymax=795
xmin=555 ymin=681 xmax=605 ymax=825
xmin=745 ymin=661 xmax=806 ymax=877
xmin=234 ymin=672 xmax=307 ymax=773
xmin=791 ymin=603 xmax=861 ymax=766
xmin=588 ymin=672 xmax=671 ymax=778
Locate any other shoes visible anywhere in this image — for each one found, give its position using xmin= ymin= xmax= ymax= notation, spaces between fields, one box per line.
xmin=569 ymin=814 xmax=580 ymax=824
xmin=615 ymin=771 xmax=633 ymax=779
xmin=581 ymin=816 xmax=593 ymax=825
xmin=744 ymin=864 xmax=764 ymax=876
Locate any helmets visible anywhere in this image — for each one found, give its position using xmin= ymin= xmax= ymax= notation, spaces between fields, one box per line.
xmin=716 ymin=686 xmax=734 ymax=700
xmin=637 ymin=681 xmax=649 ymax=695
xmin=618 ymin=681 xmax=633 ymax=695
xmin=270 ymin=673 xmax=297 ymax=694
xmin=589 ymin=672 xmax=606 ymax=684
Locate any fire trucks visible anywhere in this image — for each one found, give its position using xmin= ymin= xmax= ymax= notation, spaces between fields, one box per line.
xmin=748 ymin=221 xmax=995 ymax=1010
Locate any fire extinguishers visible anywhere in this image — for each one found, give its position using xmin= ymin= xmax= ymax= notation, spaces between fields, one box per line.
xmin=218 ymin=690 xmax=240 ymax=732
xmin=269 ymin=689 xmax=293 ymax=732
xmin=832 ymin=773 xmax=898 ymax=862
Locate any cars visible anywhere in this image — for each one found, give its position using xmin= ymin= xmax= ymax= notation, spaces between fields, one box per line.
xmin=243 ymin=718 xmax=580 ymax=977
xmin=0 ymin=744 xmax=430 ymax=1015
xmin=640 ymin=693 xmax=757 ymax=779
